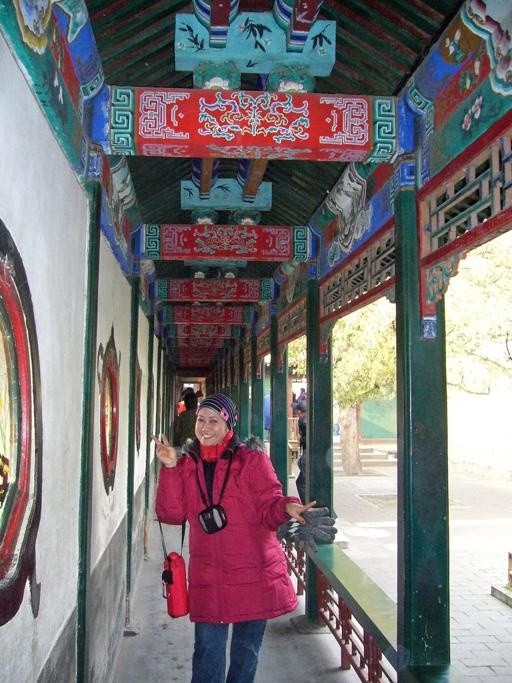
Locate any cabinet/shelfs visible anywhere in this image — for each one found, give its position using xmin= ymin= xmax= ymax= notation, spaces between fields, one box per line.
xmin=162 ymin=551 xmax=190 ymax=617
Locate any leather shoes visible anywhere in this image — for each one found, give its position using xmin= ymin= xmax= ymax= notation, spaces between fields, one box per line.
xmin=276 ymin=507 xmax=338 ymax=552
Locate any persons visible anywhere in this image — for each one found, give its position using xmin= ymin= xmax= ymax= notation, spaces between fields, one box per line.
xmin=263 ymin=395 xmax=271 ymax=441
xmin=150 ymin=395 xmax=319 ymax=683
xmin=293 ymin=388 xmax=309 ymax=455
xmin=174 ymin=388 xmax=203 ymax=447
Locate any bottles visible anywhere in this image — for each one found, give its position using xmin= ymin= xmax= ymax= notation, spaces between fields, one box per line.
xmin=196 ymin=394 xmax=238 ymax=430
